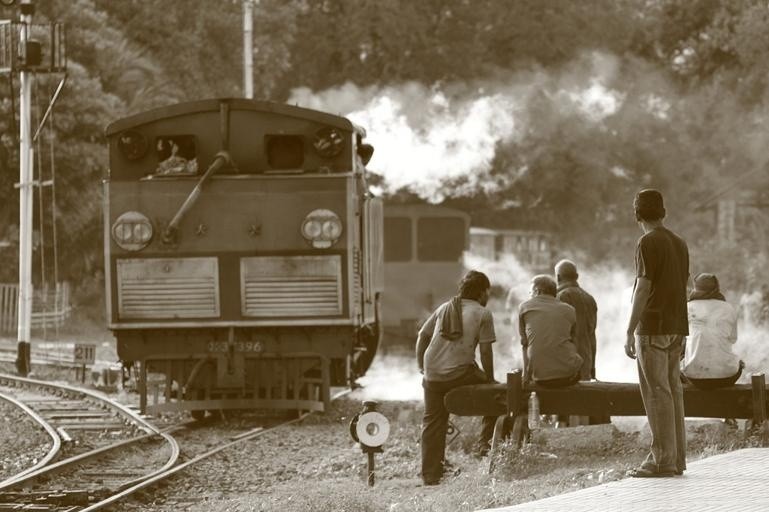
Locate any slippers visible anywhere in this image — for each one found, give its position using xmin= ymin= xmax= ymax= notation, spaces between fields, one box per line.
xmin=627 ymin=466 xmax=683 ymax=478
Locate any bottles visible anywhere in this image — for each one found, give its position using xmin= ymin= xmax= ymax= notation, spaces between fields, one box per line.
xmin=526 ymin=391 xmax=541 ymax=430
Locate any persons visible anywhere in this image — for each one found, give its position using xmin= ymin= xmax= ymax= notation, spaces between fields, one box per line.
xmin=551 ymin=257 xmax=598 ymax=432
xmin=517 ymin=274 xmax=586 ymax=424
xmin=624 ymin=188 xmax=691 ymax=477
xmin=414 ymin=269 xmax=507 ymax=485
xmin=679 ymin=272 xmax=745 ymax=428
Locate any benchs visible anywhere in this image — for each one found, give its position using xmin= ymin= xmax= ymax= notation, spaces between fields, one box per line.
xmin=442 ymin=371 xmax=767 ymax=479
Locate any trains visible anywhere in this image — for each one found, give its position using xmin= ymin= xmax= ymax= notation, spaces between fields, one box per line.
xmin=102 ymin=99 xmax=385 ymax=418
xmin=379 ymin=205 xmax=472 ymax=339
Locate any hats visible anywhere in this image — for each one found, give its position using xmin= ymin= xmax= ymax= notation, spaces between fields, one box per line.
xmin=693 ymin=272 xmax=720 ymax=291
